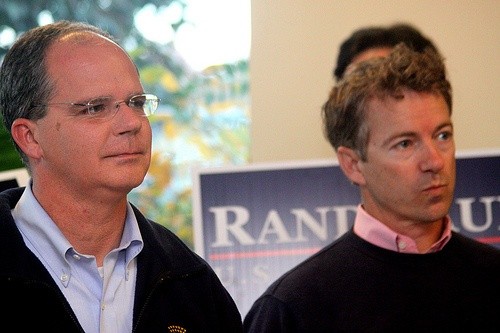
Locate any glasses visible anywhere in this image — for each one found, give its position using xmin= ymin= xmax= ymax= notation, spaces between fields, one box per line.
xmin=30 ymin=93 xmax=161 ymax=121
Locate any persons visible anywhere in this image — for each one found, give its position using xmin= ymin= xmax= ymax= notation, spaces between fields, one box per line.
xmin=243 ymin=22 xmax=500 ymax=333
xmin=0 ymin=21 xmax=243 ymax=333
